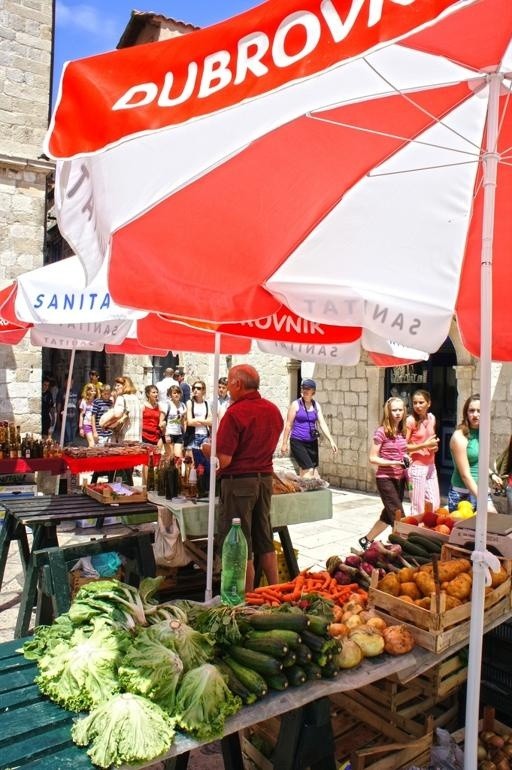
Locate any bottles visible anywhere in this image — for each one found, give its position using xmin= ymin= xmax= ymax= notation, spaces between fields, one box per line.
xmin=219 ymin=517 xmax=248 ymax=606
xmin=0 ymin=421 xmax=62 ymax=459
xmin=147 ymin=452 xmax=200 ymax=500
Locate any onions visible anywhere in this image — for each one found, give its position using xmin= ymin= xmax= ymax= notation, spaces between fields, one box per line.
xmin=326 ymin=593 xmax=416 ymax=669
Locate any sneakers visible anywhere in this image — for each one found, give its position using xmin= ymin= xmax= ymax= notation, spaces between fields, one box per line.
xmin=358 ymin=536 xmax=374 ymax=550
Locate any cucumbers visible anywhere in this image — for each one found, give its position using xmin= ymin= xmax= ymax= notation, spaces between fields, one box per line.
xmin=389 ymin=532 xmax=442 ymax=566
xmin=210 ymin=612 xmax=343 ymax=704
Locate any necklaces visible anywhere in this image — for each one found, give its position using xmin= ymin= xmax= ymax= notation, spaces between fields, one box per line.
xmin=420 ymin=416 xmax=432 ymax=430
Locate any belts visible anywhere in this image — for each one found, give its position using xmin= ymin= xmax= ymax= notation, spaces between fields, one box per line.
xmin=451 ymin=486 xmax=468 ymax=494
xmin=221 ymin=473 xmax=272 ymax=479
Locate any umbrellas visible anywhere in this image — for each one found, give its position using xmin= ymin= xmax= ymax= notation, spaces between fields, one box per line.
xmin=0 ymin=255 xmax=429 ymax=607
xmin=46 ymin=2 xmax=512 ymax=768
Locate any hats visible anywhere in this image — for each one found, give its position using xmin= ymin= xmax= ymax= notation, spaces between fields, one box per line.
xmin=300 ymin=379 xmax=316 ymax=388
xmin=173 ymin=370 xmax=184 ymax=376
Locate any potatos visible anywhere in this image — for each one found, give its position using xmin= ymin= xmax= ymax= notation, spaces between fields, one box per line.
xmin=378 ymin=558 xmax=507 ymax=631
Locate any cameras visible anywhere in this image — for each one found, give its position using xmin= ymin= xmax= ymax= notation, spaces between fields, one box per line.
xmin=401 ymin=458 xmax=409 ymax=469
xmin=309 ymin=429 xmax=320 ymax=438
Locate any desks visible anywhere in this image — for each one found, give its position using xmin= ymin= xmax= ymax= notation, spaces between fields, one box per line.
xmin=0 ymin=441 xmax=512 ymax=770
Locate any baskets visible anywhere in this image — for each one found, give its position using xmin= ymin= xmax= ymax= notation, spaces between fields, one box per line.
xmin=259 ymin=540 xmax=300 ymax=586
xmin=459 ymin=621 xmax=512 ymax=728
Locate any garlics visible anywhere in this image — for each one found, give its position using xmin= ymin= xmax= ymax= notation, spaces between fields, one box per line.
xmin=326 ymin=540 xmax=416 ymax=585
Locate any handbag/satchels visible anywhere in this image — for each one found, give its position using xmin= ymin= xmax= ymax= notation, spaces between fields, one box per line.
xmin=99 ymin=395 xmax=129 ymax=433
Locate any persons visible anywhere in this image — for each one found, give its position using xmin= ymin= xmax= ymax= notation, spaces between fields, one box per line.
xmin=498 ymin=431 xmax=512 ymax=517
xmin=404 ymin=389 xmax=441 ymax=516
xmin=43 ymin=367 xmax=234 ymax=503
xmin=279 ymin=379 xmax=338 ymax=479
xmin=216 ymin=364 xmax=284 ymax=590
xmin=358 ymin=396 xmax=413 ymax=550
xmin=447 ymin=392 xmax=503 ymax=516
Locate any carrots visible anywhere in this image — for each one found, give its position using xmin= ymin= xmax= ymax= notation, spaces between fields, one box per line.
xmin=245 ymin=568 xmax=368 ymax=607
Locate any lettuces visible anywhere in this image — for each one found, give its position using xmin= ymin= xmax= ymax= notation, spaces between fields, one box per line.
xmin=20 ymin=576 xmax=243 ymax=770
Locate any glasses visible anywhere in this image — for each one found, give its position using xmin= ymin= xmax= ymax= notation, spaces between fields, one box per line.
xmin=177 ymin=411 xmax=181 ymax=419
xmin=193 ymin=386 xmax=201 ymax=391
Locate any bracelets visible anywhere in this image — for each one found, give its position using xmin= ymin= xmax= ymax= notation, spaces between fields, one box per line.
xmin=486 ymin=497 xmax=492 ymax=502
xmin=489 ymin=472 xmax=496 ymax=480
